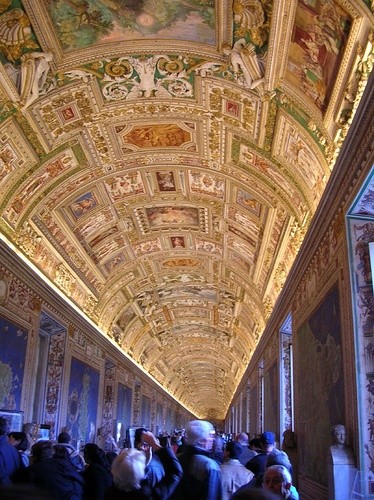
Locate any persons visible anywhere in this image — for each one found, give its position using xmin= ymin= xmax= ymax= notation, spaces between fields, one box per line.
xmin=0 ymin=418 xmax=299 ymax=500
xmin=327 ymin=424 xmax=356 ymax=465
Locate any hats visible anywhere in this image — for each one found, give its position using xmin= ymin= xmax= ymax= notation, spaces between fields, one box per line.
xmin=236 ymin=431 xmax=249 ymax=447
xmin=260 ymin=432 xmax=275 ymax=444
